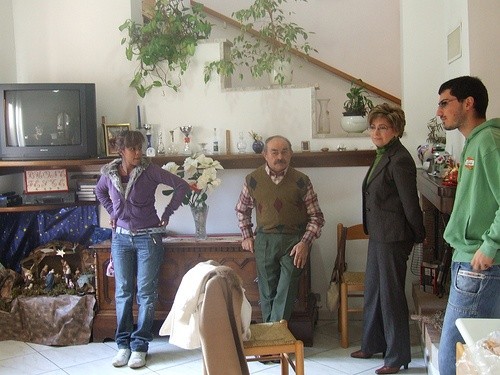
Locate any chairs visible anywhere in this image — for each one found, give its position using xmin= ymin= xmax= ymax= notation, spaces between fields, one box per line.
xmin=336 ymin=222 xmax=369 ymax=348
xmin=194 ymin=260 xmax=305 ymax=375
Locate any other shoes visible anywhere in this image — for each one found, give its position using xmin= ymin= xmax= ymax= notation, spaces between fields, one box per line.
xmin=255 ymin=354 xmax=270 ymax=364
xmin=271 ymin=353 xmax=280 ymax=363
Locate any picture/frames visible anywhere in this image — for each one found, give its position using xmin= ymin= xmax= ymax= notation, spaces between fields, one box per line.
xmin=103 ymin=123 xmax=130 ymax=157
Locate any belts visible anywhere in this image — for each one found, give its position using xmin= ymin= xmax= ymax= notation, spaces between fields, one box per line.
xmin=116 ymin=226 xmax=167 ymax=235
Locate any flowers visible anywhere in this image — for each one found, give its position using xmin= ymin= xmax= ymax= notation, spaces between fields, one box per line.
xmin=161 ymin=153 xmax=224 ymax=234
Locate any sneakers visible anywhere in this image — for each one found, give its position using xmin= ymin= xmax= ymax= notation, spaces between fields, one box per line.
xmin=128 ymin=351 xmax=146 ymax=368
xmin=112 ymin=349 xmax=130 ymax=367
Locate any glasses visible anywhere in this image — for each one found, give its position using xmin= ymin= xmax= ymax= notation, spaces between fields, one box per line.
xmin=367 ymin=124 xmax=391 ymax=131
xmin=439 ymin=97 xmax=468 ymax=108
xmin=267 ymin=151 xmax=291 ymax=157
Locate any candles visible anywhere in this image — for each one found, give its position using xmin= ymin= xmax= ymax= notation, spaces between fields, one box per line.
xmin=138 ymin=105 xmax=141 ymax=127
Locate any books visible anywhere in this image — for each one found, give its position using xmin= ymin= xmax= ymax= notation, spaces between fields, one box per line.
xmin=76 ymin=178 xmax=97 ymax=202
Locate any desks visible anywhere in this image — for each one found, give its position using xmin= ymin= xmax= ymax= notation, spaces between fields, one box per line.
xmin=455 ymin=318 xmax=500 ymax=375
xmin=412 ymin=281 xmax=448 ymax=330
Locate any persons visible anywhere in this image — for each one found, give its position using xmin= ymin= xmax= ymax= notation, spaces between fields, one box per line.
xmin=436 ymin=75 xmax=500 ymax=375
xmin=350 ymin=103 xmax=426 ymax=375
xmin=234 ymin=136 xmax=326 ymax=363
xmin=94 ymin=130 xmax=190 ymax=368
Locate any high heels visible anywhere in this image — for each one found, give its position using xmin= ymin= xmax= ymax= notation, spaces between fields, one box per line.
xmin=375 ymin=362 xmax=408 ymax=374
xmin=351 ymin=349 xmax=386 ymax=359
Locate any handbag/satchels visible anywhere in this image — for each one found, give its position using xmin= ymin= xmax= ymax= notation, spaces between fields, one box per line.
xmin=326 ymin=278 xmax=340 ymax=312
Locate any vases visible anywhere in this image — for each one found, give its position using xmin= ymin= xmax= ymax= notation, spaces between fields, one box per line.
xmin=316 ymin=98 xmax=331 ymax=138
xmin=191 ymin=208 xmax=208 ymax=240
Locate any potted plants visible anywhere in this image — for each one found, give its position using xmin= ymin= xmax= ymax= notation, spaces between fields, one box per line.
xmin=341 ymin=78 xmax=375 ymax=133
xmin=204 ymin=0 xmax=319 ymax=85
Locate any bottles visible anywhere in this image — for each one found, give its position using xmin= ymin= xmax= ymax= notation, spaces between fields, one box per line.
xmin=157 ymin=125 xmax=191 ymax=155
xmin=318 ymin=99 xmax=330 ymax=134
xmin=237 ymin=137 xmax=246 ymax=154
xmin=213 ymin=128 xmax=219 ymax=154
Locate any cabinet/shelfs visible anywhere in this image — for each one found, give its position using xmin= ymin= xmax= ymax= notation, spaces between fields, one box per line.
xmin=89 ymin=235 xmax=316 ymax=346
xmin=0 ymin=137 xmax=115 ymax=213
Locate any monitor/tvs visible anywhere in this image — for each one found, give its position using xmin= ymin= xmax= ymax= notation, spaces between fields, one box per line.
xmin=0 ymin=84 xmax=97 ymax=161
